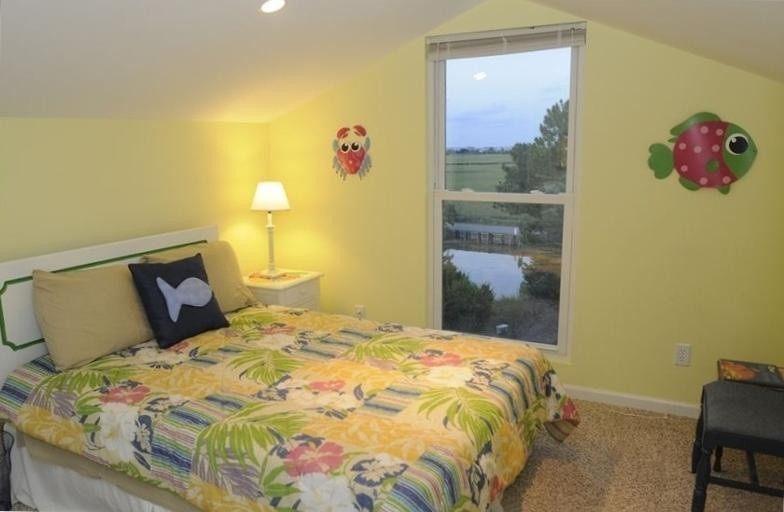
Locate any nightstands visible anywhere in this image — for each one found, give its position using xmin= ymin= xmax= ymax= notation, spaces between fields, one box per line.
xmin=242 ymin=267 xmax=326 ymax=312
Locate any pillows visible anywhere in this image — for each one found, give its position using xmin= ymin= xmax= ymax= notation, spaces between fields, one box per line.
xmin=31 ymin=256 xmax=170 ymax=374
xmin=139 ymin=239 xmax=258 ymax=316
xmin=126 ymin=252 xmax=231 ymax=349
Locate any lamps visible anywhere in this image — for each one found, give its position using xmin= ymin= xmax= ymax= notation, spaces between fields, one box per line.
xmin=252 ymin=180 xmax=292 ymax=278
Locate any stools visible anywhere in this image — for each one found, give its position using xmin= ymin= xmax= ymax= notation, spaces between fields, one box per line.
xmin=690 ymin=379 xmax=784 ymax=512
xmin=713 ymin=357 xmax=784 ymax=488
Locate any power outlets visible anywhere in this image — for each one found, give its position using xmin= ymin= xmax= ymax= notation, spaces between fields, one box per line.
xmin=353 ymin=304 xmax=366 ymax=318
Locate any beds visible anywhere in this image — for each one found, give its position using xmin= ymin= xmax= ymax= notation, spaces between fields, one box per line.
xmin=0 ymin=224 xmax=580 ymax=512
